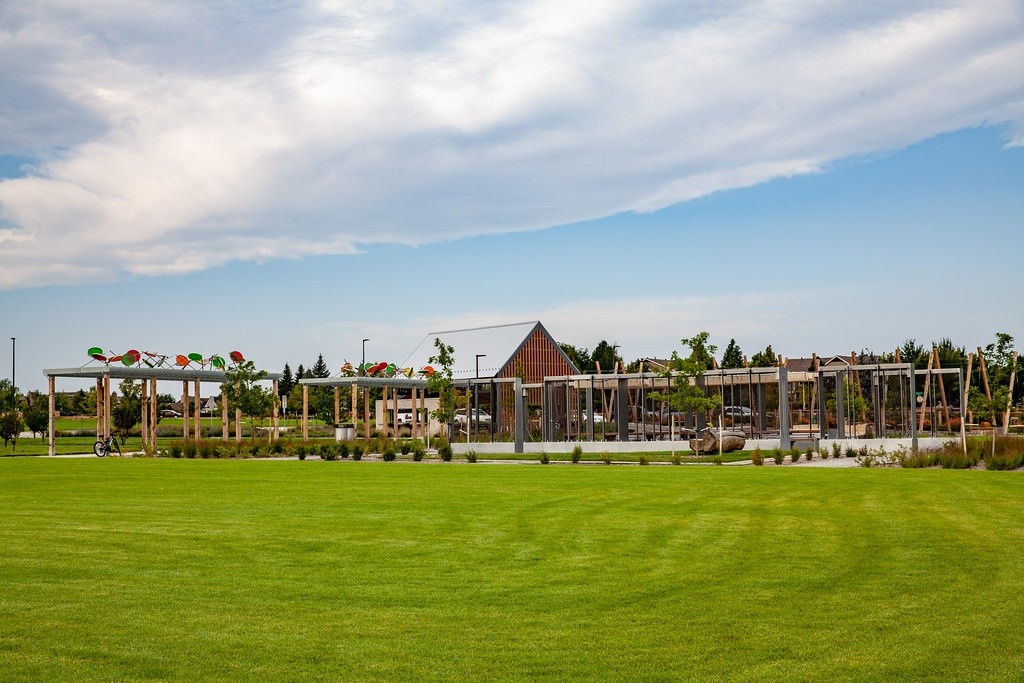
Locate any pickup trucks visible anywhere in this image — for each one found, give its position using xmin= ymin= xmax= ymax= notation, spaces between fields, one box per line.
xmin=397 ymin=413 xmax=412 ymax=422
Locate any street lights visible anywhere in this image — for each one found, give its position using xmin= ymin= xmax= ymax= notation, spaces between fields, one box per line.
xmin=476 ymin=354 xmax=487 ymax=436
xmin=362 ymin=339 xmax=370 ymax=422
xmin=10 ymin=337 xmax=16 ymax=410
xmin=613 ymin=346 xmax=622 ymax=373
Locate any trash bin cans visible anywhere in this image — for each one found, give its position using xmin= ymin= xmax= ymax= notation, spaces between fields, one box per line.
xmin=335 ymin=423 xmax=347 ymax=441
xmin=347 ymin=423 xmax=355 ymax=442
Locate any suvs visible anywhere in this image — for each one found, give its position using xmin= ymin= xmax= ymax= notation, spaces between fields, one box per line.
xmin=454 ymin=408 xmax=491 ymax=423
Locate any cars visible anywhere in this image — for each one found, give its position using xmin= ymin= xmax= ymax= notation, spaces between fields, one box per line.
xmin=654 ymin=406 xmax=684 ymax=424
xmin=723 ymin=406 xmax=758 ymax=418
xmin=569 ymin=410 xmax=607 ymax=423
xmin=630 ymin=406 xmax=652 ymax=419
xmin=160 ymin=410 xmax=182 ymax=419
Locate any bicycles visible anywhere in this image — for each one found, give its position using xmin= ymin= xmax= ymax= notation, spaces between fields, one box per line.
xmin=94 ymin=430 xmax=122 ymax=457
